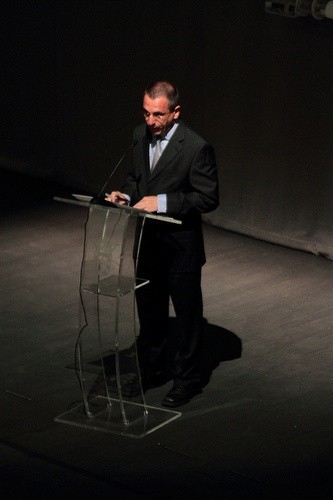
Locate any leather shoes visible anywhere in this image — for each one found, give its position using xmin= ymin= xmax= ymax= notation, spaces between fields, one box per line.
xmin=121 ymin=373 xmax=165 ymax=396
xmin=162 ymin=385 xmax=200 ymax=406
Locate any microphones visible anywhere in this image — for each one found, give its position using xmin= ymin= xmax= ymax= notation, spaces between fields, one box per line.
xmin=90 ymin=139 xmax=138 ymax=208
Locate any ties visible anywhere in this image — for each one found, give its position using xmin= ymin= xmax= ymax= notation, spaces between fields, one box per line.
xmin=151 ymin=137 xmax=162 ymax=168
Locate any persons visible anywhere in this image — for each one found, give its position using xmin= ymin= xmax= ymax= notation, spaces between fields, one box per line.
xmin=105 ymin=82 xmax=220 ymax=407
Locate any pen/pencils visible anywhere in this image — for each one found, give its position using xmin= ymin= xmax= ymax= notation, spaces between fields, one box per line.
xmin=105 ymin=192 xmax=130 ymax=202
